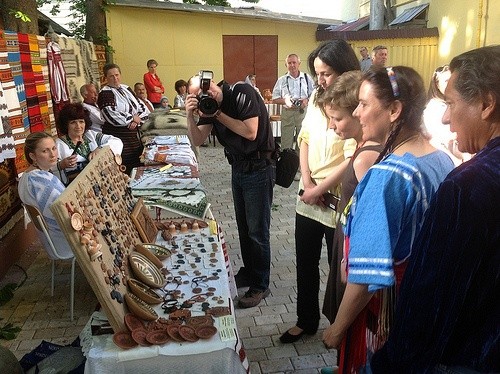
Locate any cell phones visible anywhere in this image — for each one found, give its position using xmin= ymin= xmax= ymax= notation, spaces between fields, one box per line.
xmin=298 ymin=189 xmax=304 ymax=196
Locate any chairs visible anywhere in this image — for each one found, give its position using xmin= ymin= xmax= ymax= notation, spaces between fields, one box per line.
xmin=21 ymin=202 xmax=77 ymax=323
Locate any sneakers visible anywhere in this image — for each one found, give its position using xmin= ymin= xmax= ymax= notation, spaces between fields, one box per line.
xmin=238 ymin=287 xmax=271 ymax=308
xmin=234 ymin=274 xmax=249 ymax=288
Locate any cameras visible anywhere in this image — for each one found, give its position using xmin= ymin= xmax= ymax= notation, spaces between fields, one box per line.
xmin=197 ymin=70 xmax=218 ymax=114
xmin=292 ymin=98 xmax=302 ymax=107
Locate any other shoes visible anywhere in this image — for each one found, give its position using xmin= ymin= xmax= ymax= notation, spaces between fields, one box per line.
xmin=320 ymin=366 xmax=339 ymax=374
xmin=279 ymin=324 xmax=319 ymax=343
xmin=201 ymin=142 xmax=208 ymax=147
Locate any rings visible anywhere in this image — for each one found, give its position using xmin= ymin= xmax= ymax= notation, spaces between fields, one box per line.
xmin=69 ymin=162 xmax=72 ymax=166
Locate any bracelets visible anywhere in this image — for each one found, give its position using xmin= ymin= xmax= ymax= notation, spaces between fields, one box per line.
xmin=214 ymin=109 xmax=222 ymax=118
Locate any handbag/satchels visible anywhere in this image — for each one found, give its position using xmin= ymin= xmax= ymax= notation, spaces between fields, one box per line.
xmin=275 ymin=147 xmax=299 ymax=188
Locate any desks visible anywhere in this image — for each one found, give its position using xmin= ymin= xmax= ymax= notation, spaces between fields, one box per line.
xmin=264 ymin=99 xmax=296 ymax=146
xmin=78 ymin=128 xmax=252 ymax=374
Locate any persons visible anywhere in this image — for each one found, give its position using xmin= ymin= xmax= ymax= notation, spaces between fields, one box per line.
xmin=48 ymin=102 xmax=123 ymax=187
xmin=80 ymin=85 xmax=102 ymax=133
xmin=369 ymin=44 xmax=500 ymax=374
xmin=18 ymin=132 xmax=75 ymax=259
xmin=271 ymin=39 xmax=477 ymax=345
xmin=174 ymin=80 xmax=208 ymax=147
xmin=134 ymin=80 xmax=170 ymax=114
xmin=322 ymin=66 xmax=456 ymax=374
xmin=144 ymin=59 xmax=165 ymax=108
xmin=97 ymin=63 xmax=148 ymax=175
xmin=186 ymin=70 xmax=278 ymax=307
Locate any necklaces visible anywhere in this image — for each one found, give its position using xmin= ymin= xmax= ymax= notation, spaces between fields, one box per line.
xmin=392 ymin=135 xmax=418 ymax=153
